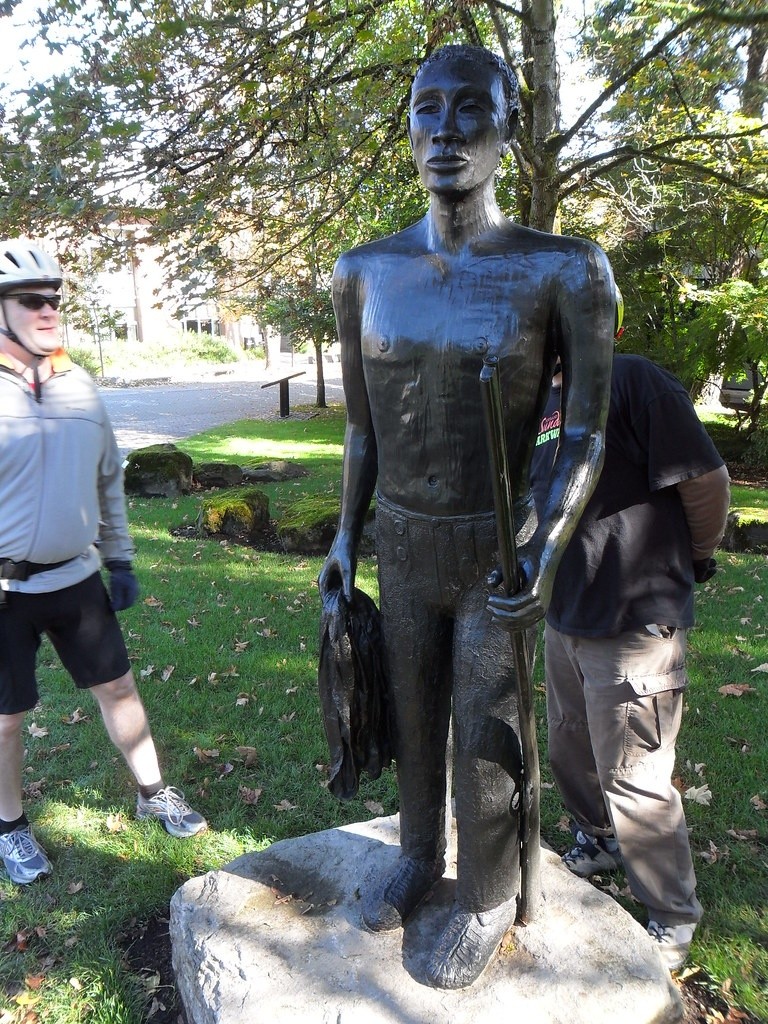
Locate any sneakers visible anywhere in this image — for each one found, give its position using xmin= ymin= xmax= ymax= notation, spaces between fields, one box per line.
xmin=0 ymin=825 xmax=53 ymax=884
xmin=646 ymin=915 xmax=698 ymax=970
xmin=136 ymin=785 xmax=207 ymax=838
xmin=560 ymin=820 xmax=624 ymax=878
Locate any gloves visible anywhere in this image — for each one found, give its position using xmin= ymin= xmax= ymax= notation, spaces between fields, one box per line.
xmin=109 ymin=567 xmax=138 ymax=612
xmin=692 ymin=557 xmax=717 ymax=583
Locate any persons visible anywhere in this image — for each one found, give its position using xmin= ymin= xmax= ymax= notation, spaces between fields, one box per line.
xmin=0 ymin=238 xmax=207 ymax=885
xmin=531 ymin=284 xmax=731 ymax=970
xmin=317 ymin=45 xmax=618 ymax=992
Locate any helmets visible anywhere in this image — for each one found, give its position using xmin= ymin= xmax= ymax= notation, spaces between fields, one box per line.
xmin=0 ymin=240 xmax=62 ymax=292
xmin=614 ymin=282 xmax=625 ymax=337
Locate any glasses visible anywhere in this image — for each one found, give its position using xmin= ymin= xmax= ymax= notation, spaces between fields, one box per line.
xmin=0 ymin=293 xmax=61 ymax=310
